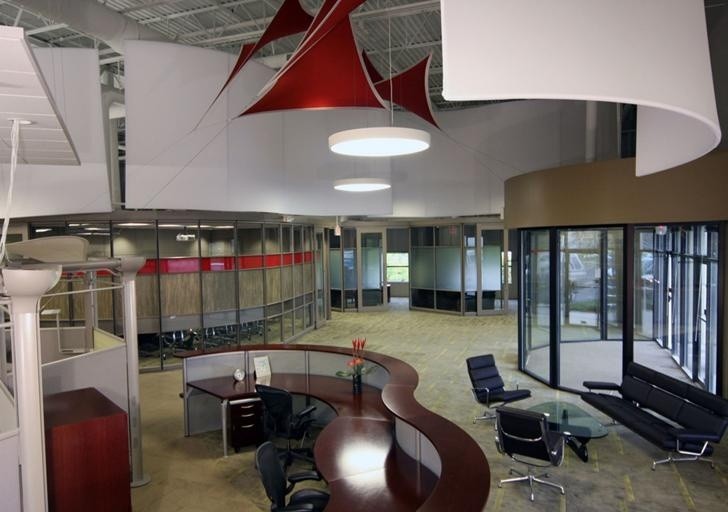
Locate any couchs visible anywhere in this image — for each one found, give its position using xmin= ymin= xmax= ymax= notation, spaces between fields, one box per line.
xmin=466 ymin=354 xmax=529 ymax=430
xmin=495 ymin=405 xmax=565 ymax=500
xmin=582 ymin=360 xmax=726 ymax=470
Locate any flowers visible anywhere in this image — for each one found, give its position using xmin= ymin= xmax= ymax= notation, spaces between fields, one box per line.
xmin=335 ymin=336 xmax=380 ymax=377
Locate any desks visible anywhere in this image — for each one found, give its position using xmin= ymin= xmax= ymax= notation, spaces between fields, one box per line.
xmin=174 ymin=343 xmax=491 ymax=512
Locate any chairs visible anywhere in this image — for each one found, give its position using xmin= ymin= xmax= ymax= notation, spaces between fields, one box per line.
xmin=255 ymin=442 xmax=330 ymax=512
xmin=255 ymin=385 xmax=318 ymax=450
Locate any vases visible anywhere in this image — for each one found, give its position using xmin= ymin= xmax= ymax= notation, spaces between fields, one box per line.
xmin=353 ymin=374 xmax=361 ymax=393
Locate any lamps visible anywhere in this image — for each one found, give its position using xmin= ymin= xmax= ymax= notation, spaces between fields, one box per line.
xmin=326 ymin=0 xmax=430 ymax=158
xmin=332 ymin=178 xmax=392 ymax=193
